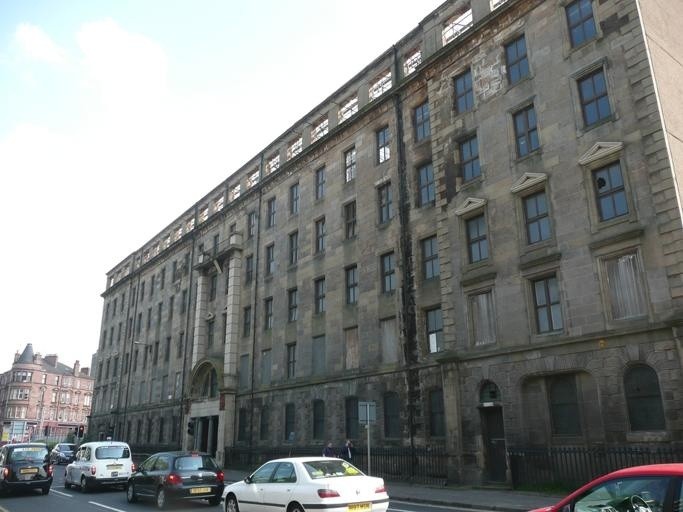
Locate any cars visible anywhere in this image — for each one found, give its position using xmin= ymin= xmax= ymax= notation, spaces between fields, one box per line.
xmin=520 ymin=462 xmax=681 ymax=511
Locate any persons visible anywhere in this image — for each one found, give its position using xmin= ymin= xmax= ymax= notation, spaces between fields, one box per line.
xmin=322 ymin=439 xmax=353 ymax=464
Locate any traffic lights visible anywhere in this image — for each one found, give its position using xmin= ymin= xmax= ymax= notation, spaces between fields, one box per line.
xmin=187 ymin=421 xmax=195 ymax=435
xmin=74 ymin=426 xmax=78 ymax=435
xmin=78 ymin=424 xmax=86 ymax=438
